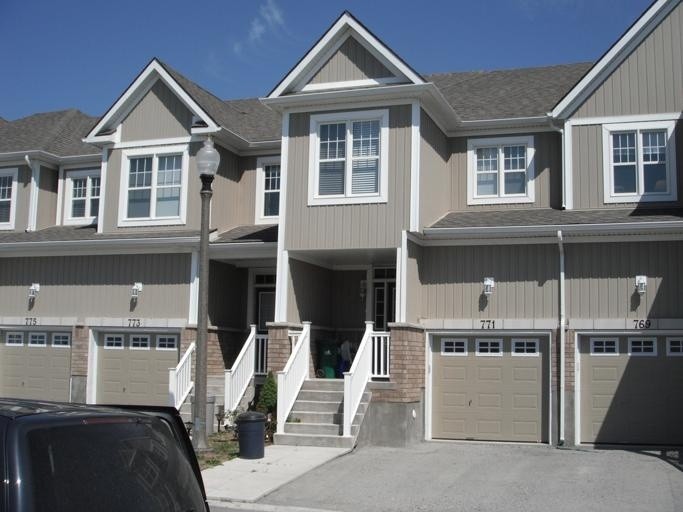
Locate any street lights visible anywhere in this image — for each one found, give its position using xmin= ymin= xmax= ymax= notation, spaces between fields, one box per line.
xmin=192 ymin=131 xmax=220 ymax=455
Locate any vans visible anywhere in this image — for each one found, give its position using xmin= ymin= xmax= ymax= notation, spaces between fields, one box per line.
xmin=1 ymin=399 xmax=208 ymax=511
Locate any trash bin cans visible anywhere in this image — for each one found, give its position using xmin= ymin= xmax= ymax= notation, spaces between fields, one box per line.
xmin=237 ymin=412 xmax=267 ymax=459
xmin=316 ymin=338 xmax=338 ymax=378
